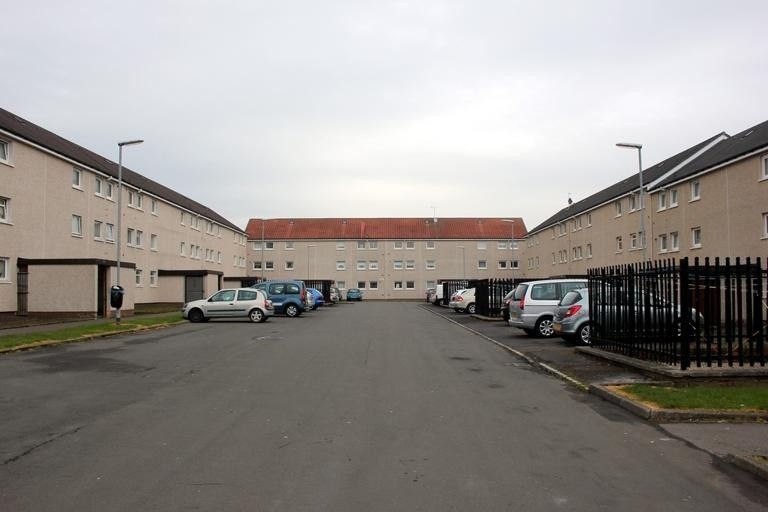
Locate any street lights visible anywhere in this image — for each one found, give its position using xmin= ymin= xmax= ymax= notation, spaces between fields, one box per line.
xmin=115 ymin=139 xmax=145 ymax=326
xmin=501 ymin=219 xmax=515 ymax=279
xmin=616 ymin=143 xmax=647 ymax=265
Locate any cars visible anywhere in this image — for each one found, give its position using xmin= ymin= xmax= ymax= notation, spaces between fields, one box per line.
xmin=346 ymin=288 xmax=363 ymax=302
xmin=426 ymin=283 xmax=512 ymax=314
xmin=275 ymin=286 xmax=342 ymax=312
xmin=550 ymin=285 xmax=706 ymax=347
xmin=182 ymin=287 xmax=276 ymax=324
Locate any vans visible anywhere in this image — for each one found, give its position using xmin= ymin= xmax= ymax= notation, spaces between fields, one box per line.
xmin=500 ymin=278 xmax=611 ymax=339
xmin=249 ymin=280 xmax=308 ymax=318
